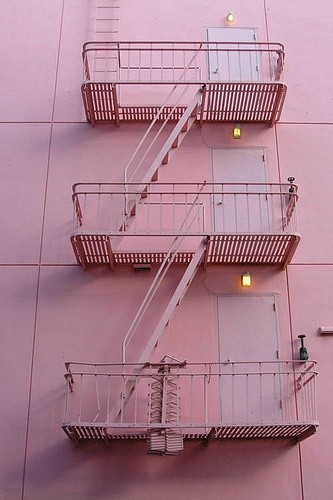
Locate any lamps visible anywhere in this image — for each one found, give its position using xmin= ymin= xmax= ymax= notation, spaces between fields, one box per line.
xmin=233 ymin=124 xmax=241 ymax=139
xmin=226 ymin=11 xmax=234 ymax=23
xmin=241 ymin=272 xmax=251 ymax=286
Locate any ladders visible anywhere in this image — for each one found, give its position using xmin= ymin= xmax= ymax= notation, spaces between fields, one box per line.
xmin=90 ymin=0 xmax=122 ymax=107
